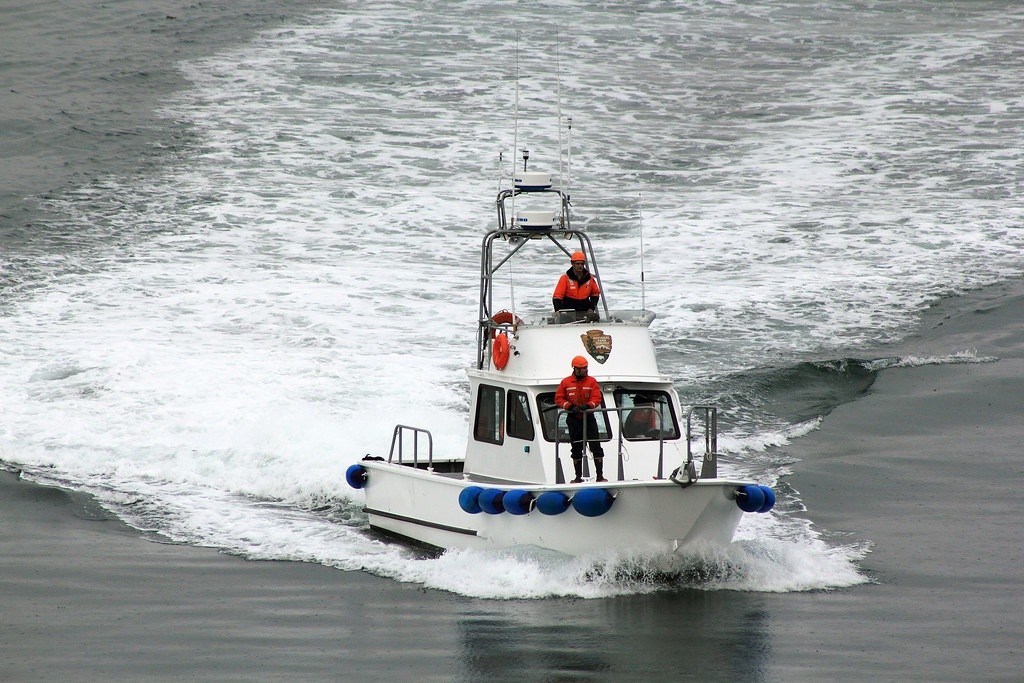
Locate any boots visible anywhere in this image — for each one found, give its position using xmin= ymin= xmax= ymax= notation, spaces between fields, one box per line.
xmin=594 ymin=457 xmax=608 ymax=482
xmin=569 ymin=459 xmax=582 ymax=484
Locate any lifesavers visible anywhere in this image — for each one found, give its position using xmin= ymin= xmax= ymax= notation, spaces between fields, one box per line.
xmin=492 ymin=332 xmax=508 ymax=370
xmin=490 ymin=311 xmax=526 ymax=339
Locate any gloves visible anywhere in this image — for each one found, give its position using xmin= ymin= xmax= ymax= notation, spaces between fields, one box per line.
xmin=569 ymin=404 xmax=579 ymax=415
xmin=554 ymin=310 xmax=561 ymax=324
xmin=588 ymin=309 xmax=593 ymax=314
xmin=581 ymin=404 xmax=590 ymax=411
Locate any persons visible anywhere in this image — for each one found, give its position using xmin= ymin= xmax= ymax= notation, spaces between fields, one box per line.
xmin=552 ymin=251 xmax=600 ymax=314
xmin=555 ymin=356 xmax=608 ymax=483
xmin=624 ymin=396 xmax=656 ymax=438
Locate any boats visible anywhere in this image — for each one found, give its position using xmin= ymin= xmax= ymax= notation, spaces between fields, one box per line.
xmin=346 ymin=21 xmax=777 ymax=583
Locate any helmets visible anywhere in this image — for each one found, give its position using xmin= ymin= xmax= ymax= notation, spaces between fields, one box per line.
xmin=572 ymin=251 xmax=586 ymax=261
xmin=572 ymin=356 xmax=589 ymax=368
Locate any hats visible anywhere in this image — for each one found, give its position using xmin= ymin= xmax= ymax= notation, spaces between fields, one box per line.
xmin=633 ymin=392 xmax=652 ymax=405
